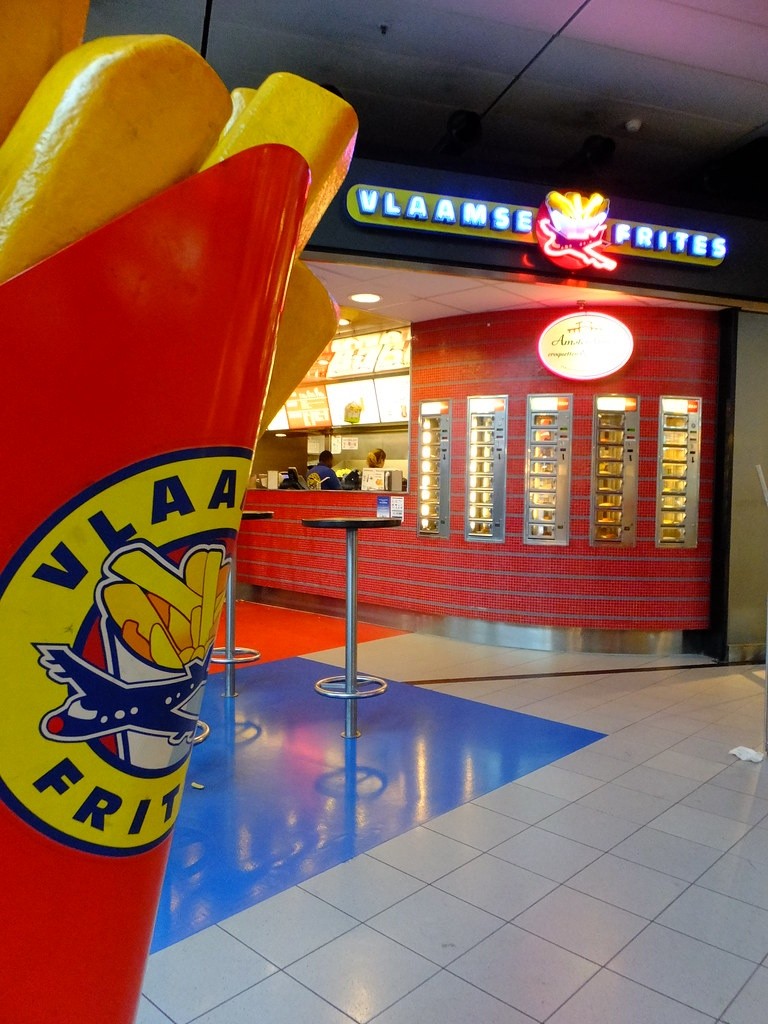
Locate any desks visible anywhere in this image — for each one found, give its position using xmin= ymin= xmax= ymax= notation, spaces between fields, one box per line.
xmin=302 ymin=519 xmax=402 ymax=739
xmin=209 ymin=510 xmax=274 ymax=698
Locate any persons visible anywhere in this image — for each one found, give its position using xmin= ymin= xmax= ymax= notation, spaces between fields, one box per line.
xmin=305 ymin=450 xmax=344 ymax=490
xmin=367 ymin=448 xmax=386 ymax=468
